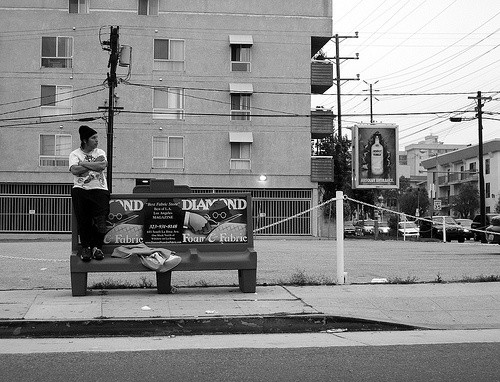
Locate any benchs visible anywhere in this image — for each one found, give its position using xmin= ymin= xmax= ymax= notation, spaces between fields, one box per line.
xmin=70 ymin=192 xmax=257 ymax=296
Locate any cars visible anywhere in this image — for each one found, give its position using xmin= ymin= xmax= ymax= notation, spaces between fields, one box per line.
xmin=344 ymin=219 xmax=391 ymax=237
xmin=455 ymin=219 xmax=473 ymax=240
xmin=398 ymin=222 xmax=420 ymax=237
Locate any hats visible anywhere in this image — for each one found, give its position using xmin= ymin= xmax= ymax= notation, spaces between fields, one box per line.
xmin=79 ymin=126 xmax=97 ymax=142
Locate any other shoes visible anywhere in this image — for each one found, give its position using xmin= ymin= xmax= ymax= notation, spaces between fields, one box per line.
xmin=81 ymin=247 xmax=92 ymax=261
xmin=92 ymin=247 xmax=104 ymax=260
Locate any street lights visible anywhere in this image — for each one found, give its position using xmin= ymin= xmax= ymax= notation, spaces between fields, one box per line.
xmin=449 ymin=116 xmax=488 ymax=244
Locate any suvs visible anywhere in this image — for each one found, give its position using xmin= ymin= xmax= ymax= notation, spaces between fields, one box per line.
xmin=470 ymin=213 xmax=500 ymax=244
xmin=419 ymin=215 xmax=470 ymax=243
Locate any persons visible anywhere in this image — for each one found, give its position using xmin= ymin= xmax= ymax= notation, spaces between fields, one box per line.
xmin=69 ymin=124 xmax=110 ymax=261
